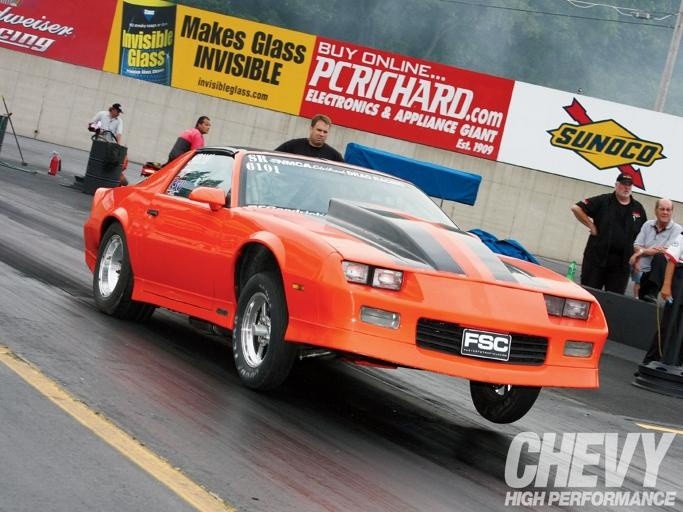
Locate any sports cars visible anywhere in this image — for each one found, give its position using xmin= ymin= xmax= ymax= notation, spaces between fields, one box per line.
xmin=84 ymin=146 xmax=609 ymax=426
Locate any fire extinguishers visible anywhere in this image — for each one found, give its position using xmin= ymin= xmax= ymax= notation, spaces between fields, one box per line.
xmin=49 ymin=151 xmax=59 ymax=175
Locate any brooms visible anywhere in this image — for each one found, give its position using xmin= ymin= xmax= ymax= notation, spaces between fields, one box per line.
xmin=1 ymin=96 xmax=47 ymax=177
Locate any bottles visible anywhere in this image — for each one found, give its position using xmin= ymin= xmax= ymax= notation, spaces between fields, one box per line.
xmin=565 ymin=259 xmax=577 ymax=281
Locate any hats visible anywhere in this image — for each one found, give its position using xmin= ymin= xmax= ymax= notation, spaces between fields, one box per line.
xmin=114 ymin=104 xmax=123 ymax=113
xmin=618 ymin=173 xmax=633 ymax=185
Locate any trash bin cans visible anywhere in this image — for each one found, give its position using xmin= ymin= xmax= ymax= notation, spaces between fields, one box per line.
xmin=0 ymin=115 xmax=8 ymax=151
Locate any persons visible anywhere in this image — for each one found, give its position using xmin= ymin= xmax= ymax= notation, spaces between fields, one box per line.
xmin=633 ymin=232 xmax=683 ymax=377
xmin=273 ymin=114 xmax=346 ymax=163
xmin=120 ymin=156 xmax=129 ymax=186
xmin=168 ymin=116 xmax=211 ymax=163
xmin=570 ymin=173 xmax=647 ymax=295
xmin=88 ymin=103 xmax=124 ymax=145
xmin=628 ymin=198 xmax=682 ymax=302
xmin=631 ymin=269 xmax=644 ymax=300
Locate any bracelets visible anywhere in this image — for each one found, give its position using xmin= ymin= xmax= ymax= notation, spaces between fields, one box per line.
xmin=638 ymin=248 xmax=644 ymax=255
